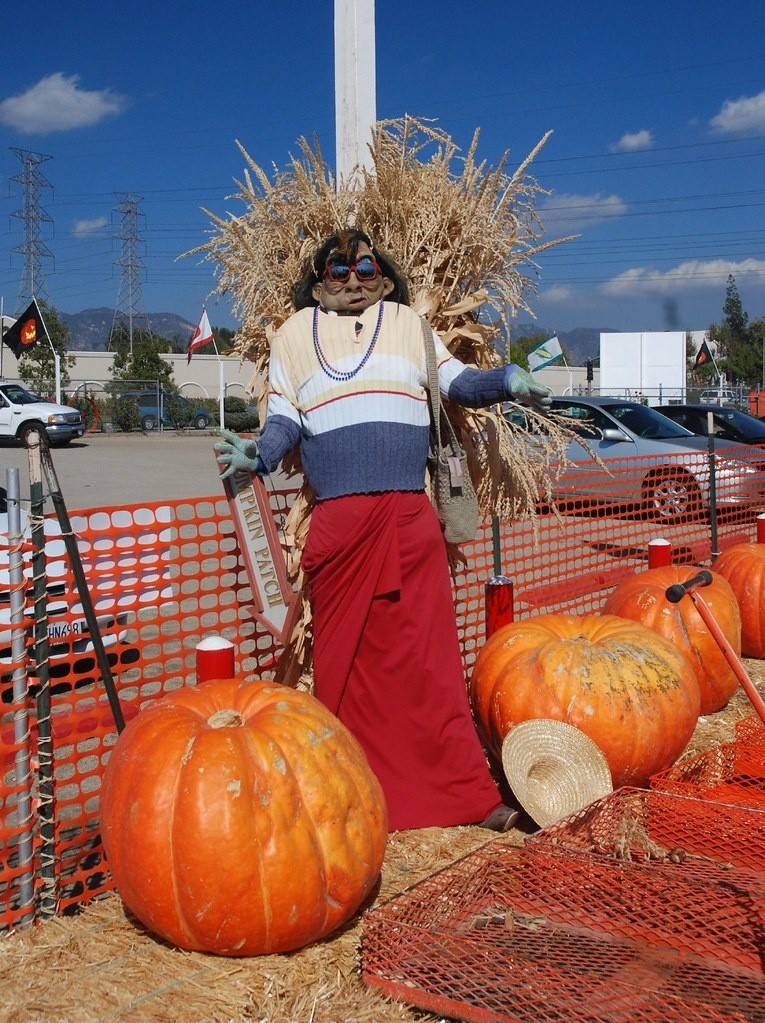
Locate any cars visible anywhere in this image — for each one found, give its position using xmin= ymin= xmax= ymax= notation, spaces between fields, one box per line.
xmin=472 ymin=396 xmax=765 ymax=525
xmin=615 ymin=404 xmax=765 ymax=445
xmin=112 ymin=393 xmax=212 ymax=429
xmin=0 ymin=382 xmax=86 ymax=449
xmin=0 ymin=485 xmax=130 ymax=679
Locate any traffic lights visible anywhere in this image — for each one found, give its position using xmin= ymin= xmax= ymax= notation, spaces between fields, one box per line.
xmin=587 ymin=361 xmax=593 ymax=380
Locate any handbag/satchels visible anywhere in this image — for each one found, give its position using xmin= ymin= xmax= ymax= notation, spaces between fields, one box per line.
xmin=419 ymin=314 xmax=479 ymax=545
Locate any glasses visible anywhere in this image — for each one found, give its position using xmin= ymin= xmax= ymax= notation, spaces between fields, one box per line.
xmin=320 ymin=262 xmax=383 ymax=281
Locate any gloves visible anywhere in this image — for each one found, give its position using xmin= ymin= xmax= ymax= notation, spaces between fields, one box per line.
xmin=506 ymin=366 xmax=553 ymax=417
xmin=214 ymin=430 xmax=261 ymax=479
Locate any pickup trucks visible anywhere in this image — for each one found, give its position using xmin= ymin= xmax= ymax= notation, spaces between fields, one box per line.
xmin=700 ymin=390 xmax=746 ymax=404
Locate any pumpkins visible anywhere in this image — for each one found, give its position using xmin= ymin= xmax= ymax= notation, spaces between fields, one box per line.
xmin=600 ymin=567 xmax=742 ymax=715
xmin=710 ymin=542 xmax=765 ymax=661
xmin=98 ymin=679 xmax=388 ymax=956
xmin=466 ymin=615 xmax=700 ymax=791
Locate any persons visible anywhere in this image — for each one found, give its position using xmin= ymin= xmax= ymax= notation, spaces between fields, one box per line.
xmin=213 ymin=230 xmax=553 ymax=831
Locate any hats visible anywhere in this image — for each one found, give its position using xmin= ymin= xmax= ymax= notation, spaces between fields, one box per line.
xmin=500 ymin=719 xmax=614 ymax=832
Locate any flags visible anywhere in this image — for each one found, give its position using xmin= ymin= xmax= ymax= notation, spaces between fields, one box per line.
xmin=690 ymin=341 xmax=713 ymax=373
xmin=524 ymin=335 xmax=563 ymax=373
xmin=186 ymin=308 xmax=214 ymax=367
xmin=2 ymin=300 xmax=46 ymax=361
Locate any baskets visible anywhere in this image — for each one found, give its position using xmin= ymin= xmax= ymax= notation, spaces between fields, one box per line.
xmin=358 ymin=714 xmax=764 ymax=1023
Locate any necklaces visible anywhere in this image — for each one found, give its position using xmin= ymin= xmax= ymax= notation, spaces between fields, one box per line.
xmin=313 ymin=300 xmax=384 ymax=380
